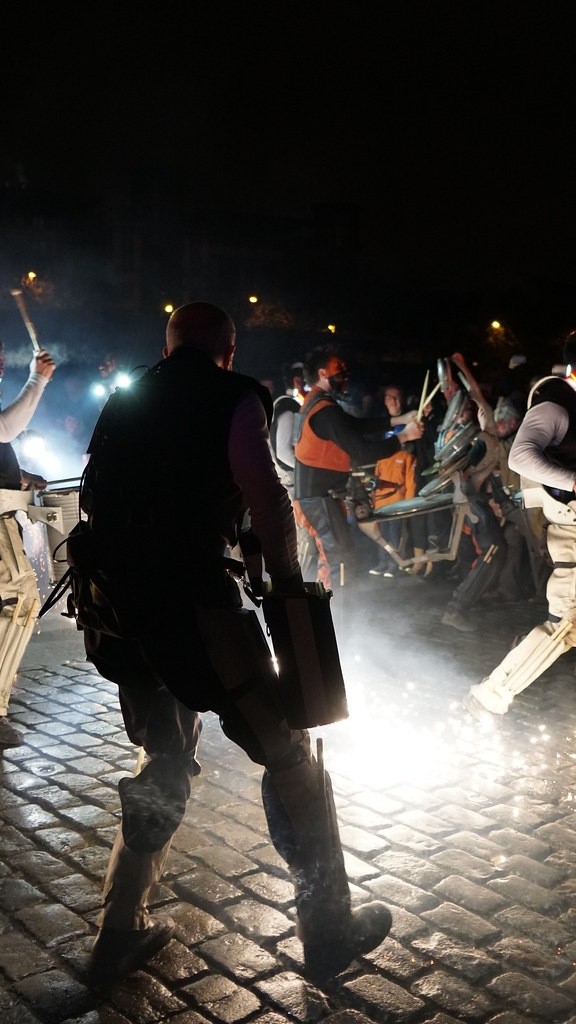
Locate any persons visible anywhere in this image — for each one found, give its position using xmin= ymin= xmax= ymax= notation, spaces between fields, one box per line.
xmin=69 ymin=302 xmax=393 ymax=983
xmin=0 ymin=272 xmax=576 ymax=748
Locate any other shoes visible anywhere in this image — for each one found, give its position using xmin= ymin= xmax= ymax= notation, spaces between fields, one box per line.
xmin=369 ymin=564 xmax=387 ymax=575
xmin=384 ymin=567 xmax=400 ymax=578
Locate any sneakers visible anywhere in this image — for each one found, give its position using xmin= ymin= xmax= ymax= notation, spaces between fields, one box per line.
xmin=302 ymin=902 xmax=392 ymax=982
xmin=483 ymin=586 xmax=517 ymax=601
xmin=440 ymin=610 xmax=478 ymax=631
xmin=0 ymin=716 xmax=24 ymax=744
xmin=90 ymin=914 xmax=175 ymax=982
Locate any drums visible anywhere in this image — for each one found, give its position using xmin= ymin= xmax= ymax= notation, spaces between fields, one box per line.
xmin=36 ymin=485 xmax=88 ymax=590
xmin=372 ymin=356 xmax=482 ymax=516
